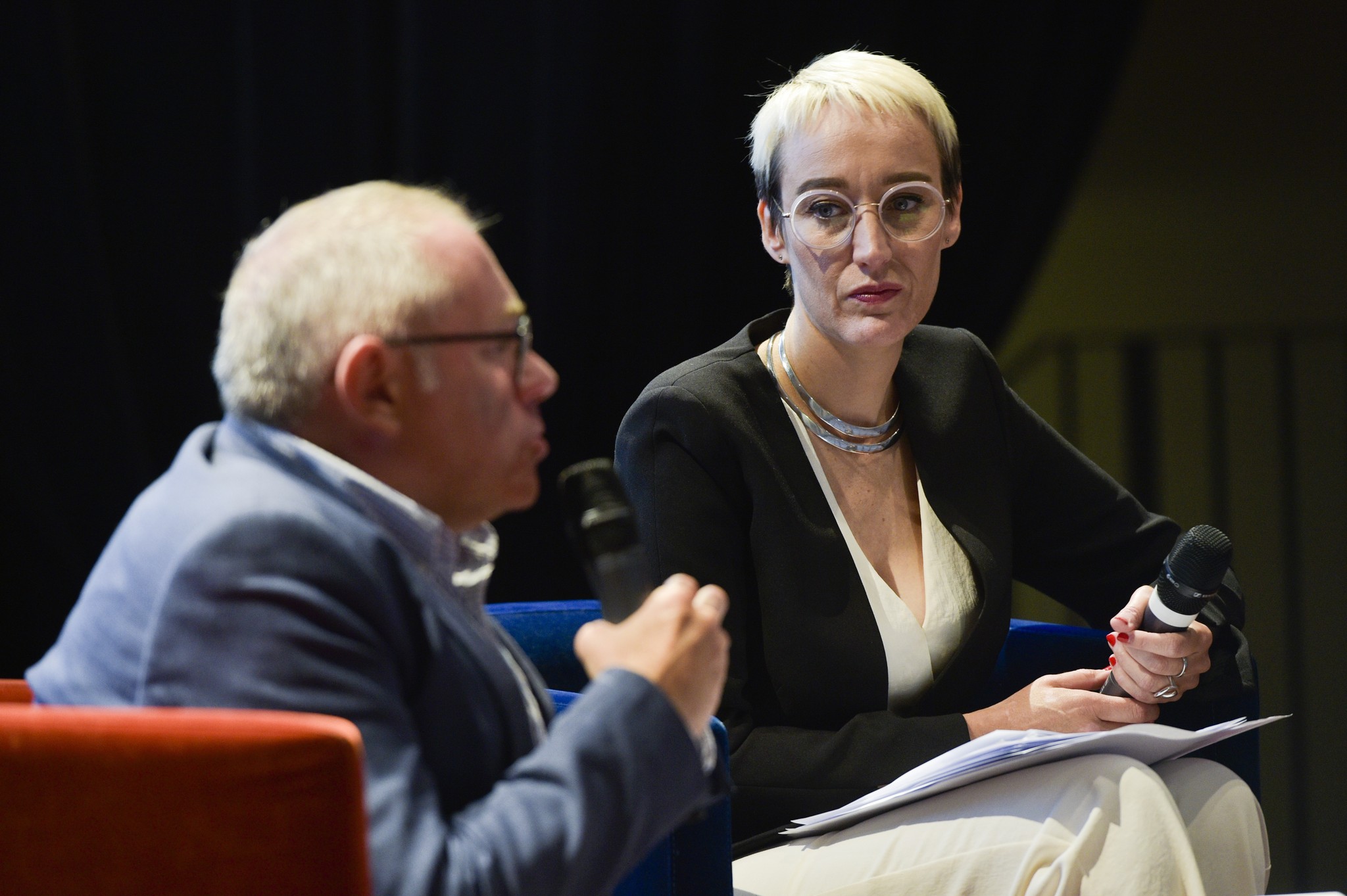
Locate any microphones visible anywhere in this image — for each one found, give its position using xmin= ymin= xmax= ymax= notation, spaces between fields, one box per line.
xmin=1099 ymin=525 xmax=1233 ymax=699
xmin=555 ymin=456 xmax=712 ymax=763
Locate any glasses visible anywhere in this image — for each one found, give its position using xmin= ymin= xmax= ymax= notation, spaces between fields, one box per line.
xmin=771 ymin=181 xmax=951 ymax=249
xmin=386 ymin=313 xmax=531 ymax=365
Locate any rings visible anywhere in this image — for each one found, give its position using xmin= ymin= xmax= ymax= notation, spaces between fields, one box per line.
xmin=1173 ymin=657 xmax=1188 ymax=677
xmin=1153 ymin=676 xmax=1179 ymax=698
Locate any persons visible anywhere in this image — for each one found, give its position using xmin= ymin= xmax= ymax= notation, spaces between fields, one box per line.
xmin=24 ymin=179 xmax=731 ymax=896
xmin=606 ymin=44 xmax=1275 ymax=896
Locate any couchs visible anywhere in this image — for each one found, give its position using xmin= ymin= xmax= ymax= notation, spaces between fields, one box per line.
xmin=486 ymin=596 xmax=1260 ymax=893
xmin=1 ymin=670 xmax=371 ymax=896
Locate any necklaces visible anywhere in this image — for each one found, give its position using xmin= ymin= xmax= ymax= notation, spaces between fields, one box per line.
xmin=766 ymin=325 xmax=905 ymax=453
